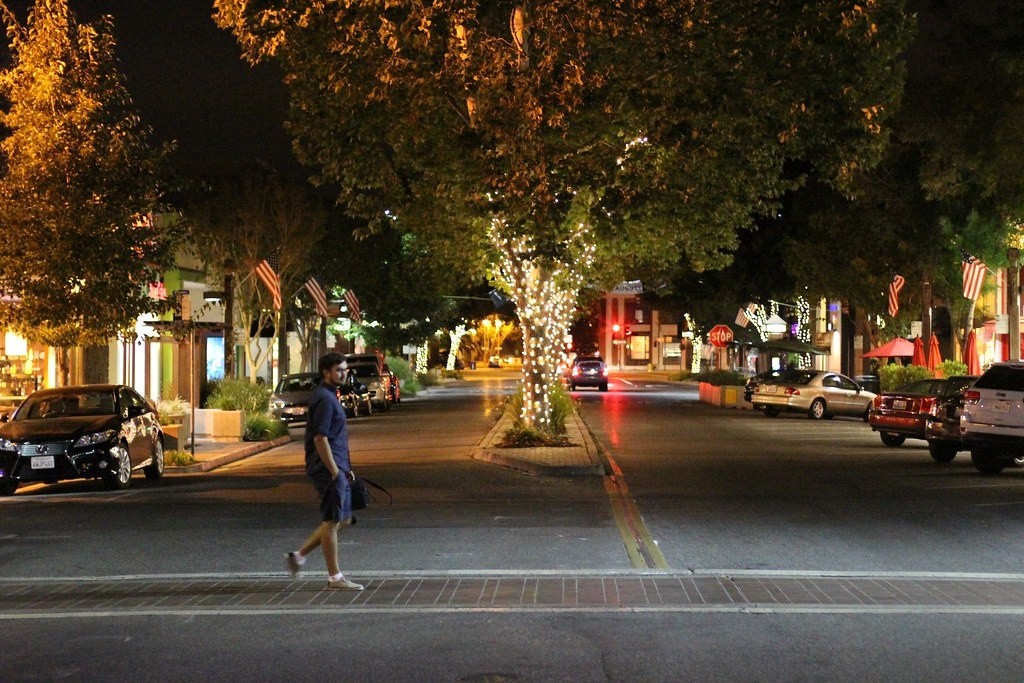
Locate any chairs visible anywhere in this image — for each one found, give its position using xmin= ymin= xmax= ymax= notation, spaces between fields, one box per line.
xmin=60 ymin=397 xmax=79 ymax=413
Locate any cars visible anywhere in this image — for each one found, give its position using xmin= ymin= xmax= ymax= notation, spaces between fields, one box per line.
xmin=488 ymin=355 xmax=503 ymax=368
xmin=865 ymin=377 xmax=949 ymax=449
xmin=267 ymin=371 xmax=320 ymax=429
xmin=743 ymin=367 xmax=880 ymax=423
xmin=336 ymin=351 xmax=401 ymax=418
xmin=922 ymin=375 xmax=981 ymax=464
xmin=566 ymin=355 xmax=608 ymax=392
xmin=0 ymin=383 xmax=165 ymax=498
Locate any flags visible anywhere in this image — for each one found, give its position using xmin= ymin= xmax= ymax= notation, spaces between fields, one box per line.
xmin=305 ymin=263 xmax=328 ymax=317
xmin=254 ymin=248 xmax=282 ymax=309
xmin=962 ymin=250 xmax=987 ymax=300
xmin=889 ymin=269 xmax=905 ymax=317
xmin=344 ymin=287 xmax=360 ymax=321
xmin=735 ymin=300 xmax=756 ymax=327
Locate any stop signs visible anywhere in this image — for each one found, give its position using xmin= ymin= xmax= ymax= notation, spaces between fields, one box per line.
xmin=709 ymin=324 xmax=734 ymax=349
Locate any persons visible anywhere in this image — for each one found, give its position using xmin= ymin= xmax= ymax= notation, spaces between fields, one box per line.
xmin=286 ymin=353 xmax=363 ymax=590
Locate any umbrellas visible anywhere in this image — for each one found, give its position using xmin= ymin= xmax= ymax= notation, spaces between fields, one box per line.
xmin=928 ymin=332 xmax=943 ymax=377
xmin=912 ymin=334 xmax=927 ymax=367
xmin=725 ymin=338 xmax=832 ymax=369
xmin=963 ymin=330 xmax=980 ymax=375
xmin=863 ymin=339 xmax=913 ymax=357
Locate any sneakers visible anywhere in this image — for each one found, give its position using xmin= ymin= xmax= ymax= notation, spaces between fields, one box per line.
xmin=327 ymin=576 xmax=363 ymax=591
xmin=284 ymin=552 xmax=304 ymax=580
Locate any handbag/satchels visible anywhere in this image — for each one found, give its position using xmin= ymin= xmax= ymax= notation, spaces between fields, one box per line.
xmin=346 ymin=476 xmax=370 ymax=510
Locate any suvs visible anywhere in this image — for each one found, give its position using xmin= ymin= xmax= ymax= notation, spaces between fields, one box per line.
xmin=959 ymin=357 xmax=1024 ymax=477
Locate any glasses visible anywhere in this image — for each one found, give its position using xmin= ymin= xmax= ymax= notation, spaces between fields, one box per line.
xmin=333 ymin=368 xmax=348 ymax=374
